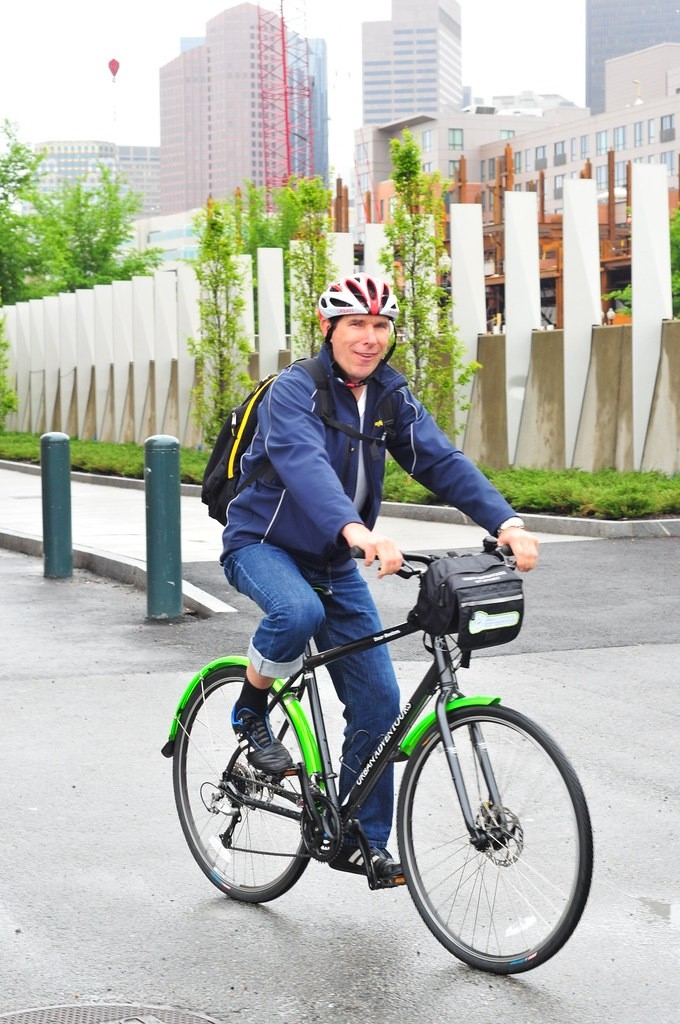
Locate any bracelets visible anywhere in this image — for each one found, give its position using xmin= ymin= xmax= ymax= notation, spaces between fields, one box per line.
xmin=498 ymin=518 xmax=525 ymax=534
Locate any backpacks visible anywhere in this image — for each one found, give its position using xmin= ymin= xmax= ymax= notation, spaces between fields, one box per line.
xmin=201 ymin=359 xmax=396 ymax=527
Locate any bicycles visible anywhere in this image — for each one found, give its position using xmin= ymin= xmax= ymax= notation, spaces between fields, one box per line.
xmin=160 ymin=533 xmax=594 ymax=975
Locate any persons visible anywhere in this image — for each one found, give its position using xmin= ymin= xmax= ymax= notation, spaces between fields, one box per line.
xmin=220 ymin=273 xmax=542 ymax=881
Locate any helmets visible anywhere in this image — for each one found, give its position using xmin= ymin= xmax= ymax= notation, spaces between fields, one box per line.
xmin=318 ymin=274 xmax=400 ymax=321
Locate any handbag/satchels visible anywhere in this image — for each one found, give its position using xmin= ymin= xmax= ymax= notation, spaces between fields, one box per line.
xmin=407 ymin=553 xmax=524 ymax=668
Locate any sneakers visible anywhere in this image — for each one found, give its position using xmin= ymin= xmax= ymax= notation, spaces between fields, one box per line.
xmin=329 ymin=840 xmax=403 ymax=878
xmin=231 ymin=700 xmax=293 ymax=773
